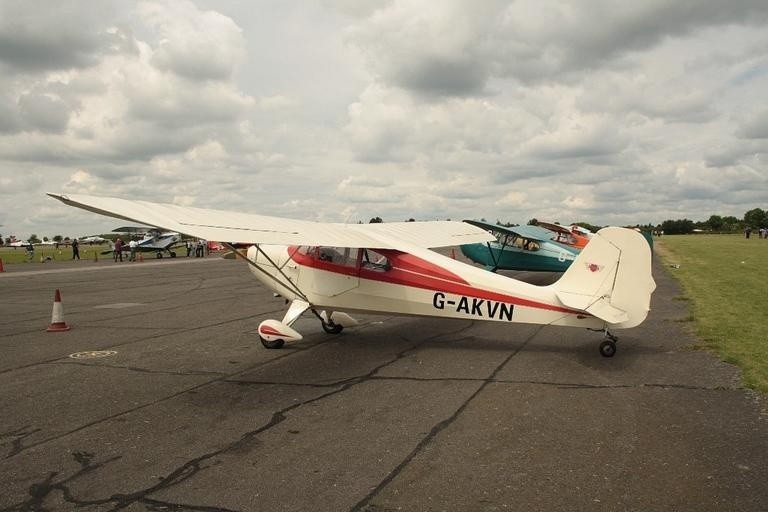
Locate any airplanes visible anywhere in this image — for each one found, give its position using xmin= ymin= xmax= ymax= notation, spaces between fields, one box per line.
xmin=460 ymin=220 xmax=577 ymax=271
xmin=3 ymin=230 xmax=200 ymax=261
xmin=531 ymin=218 xmax=596 ymax=246
xmin=44 ymin=195 xmax=657 ymax=358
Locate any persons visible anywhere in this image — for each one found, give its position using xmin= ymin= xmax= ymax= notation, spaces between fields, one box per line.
xmin=743 ymin=226 xmax=753 ymax=239
xmin=758 ymin=227 xmax=768 ymax=239
xmin=129 ymin=238 xmax=138 ymax=262
xmin=186 ymin=239 xmax=208 ymax=258
xmin=89 ymin=240 xmax=94 ymax=248
xmin=653 ymin=229 xmax=664 ymax=237
xmin=26 ymin=243 xmax=34 ymax=259
xmin=115 ymin=239 xmax=123 ymax=262
xmin=72 ymin=239 xmax=80 ymax=260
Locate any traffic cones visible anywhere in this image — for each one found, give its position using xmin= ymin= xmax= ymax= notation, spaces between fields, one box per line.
xmin=47 ymin=287 xmax=72 ymax=334
